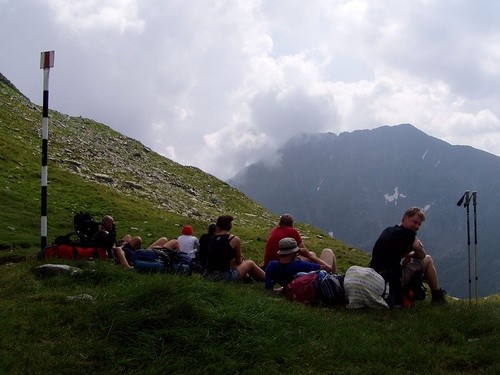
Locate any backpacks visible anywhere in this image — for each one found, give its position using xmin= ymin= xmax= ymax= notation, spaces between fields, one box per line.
xmin=343 ymin=262 xmax=391 ymax=317
xmin=285 ymin=272 xmax=342 ymax=303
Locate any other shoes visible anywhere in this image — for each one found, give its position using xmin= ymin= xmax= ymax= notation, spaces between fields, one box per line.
xmin=432 ymin=288 xmax=447 ymax=305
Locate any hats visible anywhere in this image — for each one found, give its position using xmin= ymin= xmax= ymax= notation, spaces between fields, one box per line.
xmin=277 ymin=238 xmax=299 ymax=254
xmin=182 ymin=225 xmax=192 ymax=235
